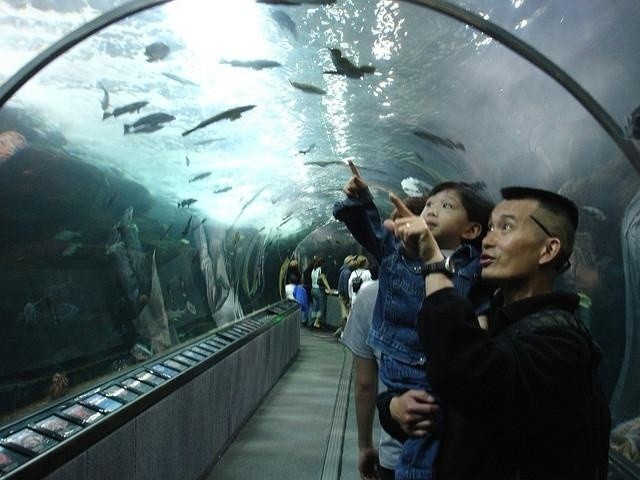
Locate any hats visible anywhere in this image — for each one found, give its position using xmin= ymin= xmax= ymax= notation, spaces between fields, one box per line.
xmin=342 ymin=254 xmax=357 ymax=266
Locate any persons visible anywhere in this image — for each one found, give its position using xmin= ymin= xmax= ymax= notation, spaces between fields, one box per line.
xmin=300 ymin=254 xmax=320 ymax=328
xmin=339 ymin=195 xmax=428 ymax=477
xmin=310 ymin=256 xmax=334 ymax=329
xmin=349 ymin=257 xmax=373 ymax=310
xmin=330 ymin=251 xmax=359 ymax=341
xmin=329 ymin=158 xmax=506 ymax=479
xmin=283 ymin=273 xmax=302 ymax=304
xmin=377 ymin=185 xmax=610 ymax=478
xmin=286 ymin=259 xmax=300 ymax=284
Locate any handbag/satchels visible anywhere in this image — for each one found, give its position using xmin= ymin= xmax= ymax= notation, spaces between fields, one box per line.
xmin=316 ymin=278 xmax=326 ymax=288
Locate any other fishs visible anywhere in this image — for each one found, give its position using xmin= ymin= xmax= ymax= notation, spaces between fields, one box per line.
xmin=176 ymin=155 xmax=234 ymax=238
xmin=49 ymin=145 xmax=126 ymax=212
xmin=292 ymin=143 xmax=346 ymax=170
xmin=267 ymin=175 xmax=347 ymax=230
xmin=55 ymin=230 xmax=83 ymax=256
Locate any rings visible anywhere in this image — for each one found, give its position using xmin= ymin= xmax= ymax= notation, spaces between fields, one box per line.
xmin=402 ymin=221 xmax=411 ymax=228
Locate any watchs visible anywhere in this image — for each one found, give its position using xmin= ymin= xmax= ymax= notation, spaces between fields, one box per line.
xmin=420 ymin=256 xmax=460 ymax=281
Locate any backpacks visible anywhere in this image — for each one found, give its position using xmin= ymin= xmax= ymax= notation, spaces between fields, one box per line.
xmin=352 ymin=269 xmax=366 ymax=293
